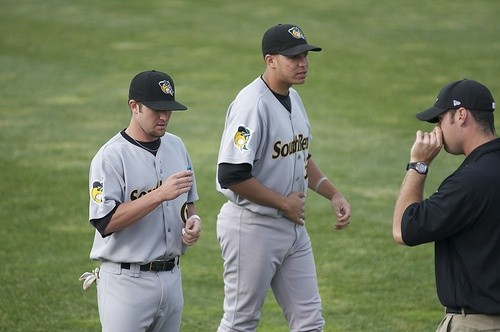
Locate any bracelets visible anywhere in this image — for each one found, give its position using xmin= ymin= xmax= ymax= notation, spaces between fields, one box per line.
xmin=315 ymin=177 xmax=328 ymax=192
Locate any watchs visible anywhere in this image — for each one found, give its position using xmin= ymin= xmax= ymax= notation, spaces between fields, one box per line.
xmin=406 ymin=161 xmax=429 ymax=175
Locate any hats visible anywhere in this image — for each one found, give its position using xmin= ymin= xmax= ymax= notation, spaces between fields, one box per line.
xmin=416 ymin=79 xmax=495 ymax=124
xmin=129 ymin=69 xmax=189 ymax=112
xmin=260 ymin=23 xmax=321 ymax=57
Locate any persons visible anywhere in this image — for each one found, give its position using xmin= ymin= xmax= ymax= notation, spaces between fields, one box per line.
xmin=79 ymin=70 xmax=200 ymax=332
xmin=393 ymin=78 xmax=500 ymax=332
xmin=216 ymin=24 xmax=351 ymax=332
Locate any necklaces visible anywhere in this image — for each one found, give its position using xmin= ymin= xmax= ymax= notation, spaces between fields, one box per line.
xmin=128 ymin=132 xmax=161 ymax=152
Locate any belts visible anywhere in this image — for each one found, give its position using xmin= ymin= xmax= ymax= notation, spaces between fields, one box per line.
xmin=122 ymin=255 xmax=180 ymax=271
xmin=444 ymin=305 xmax=478 ymax=316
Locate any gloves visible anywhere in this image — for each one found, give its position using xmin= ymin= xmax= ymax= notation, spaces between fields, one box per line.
xmin=79 ymin=268 xmax=99 ymax=290
